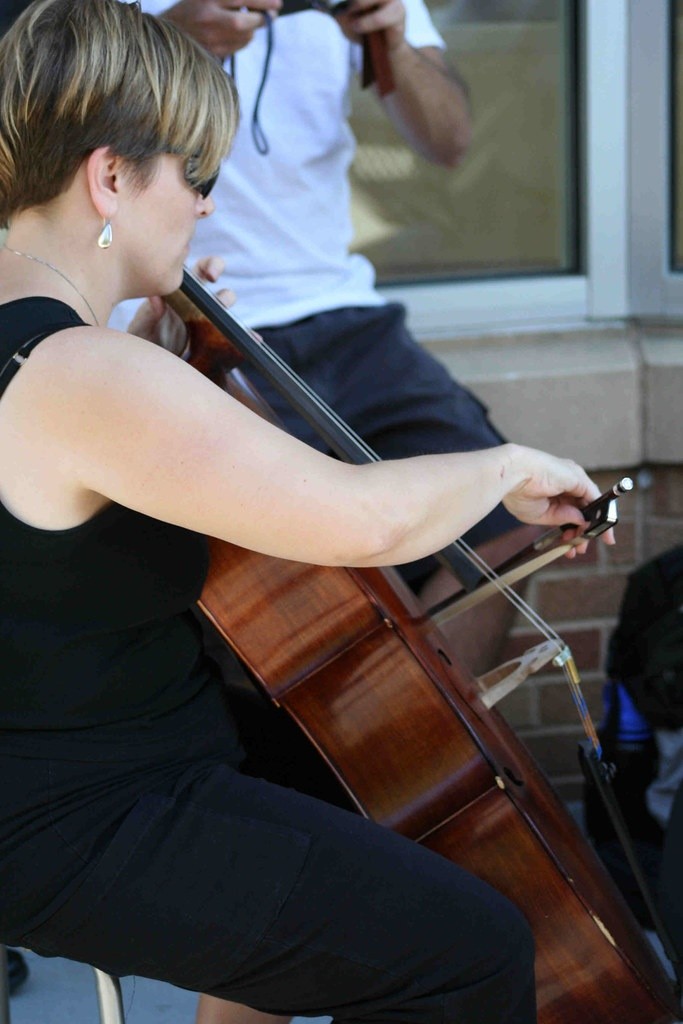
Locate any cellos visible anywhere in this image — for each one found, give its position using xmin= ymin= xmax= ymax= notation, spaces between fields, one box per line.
xmin=159 ymin=265 xmax=683 ymax=1023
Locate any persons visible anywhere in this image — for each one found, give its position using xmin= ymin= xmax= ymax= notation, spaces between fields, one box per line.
xmin=124 ymin=0 xmax=541 ymax=618
xmin=0 ymin=0 xmax=617 ymax=1024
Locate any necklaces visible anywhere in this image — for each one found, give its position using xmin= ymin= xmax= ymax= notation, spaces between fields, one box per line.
xmin=3 ymin=243 xmax=99 ymax=328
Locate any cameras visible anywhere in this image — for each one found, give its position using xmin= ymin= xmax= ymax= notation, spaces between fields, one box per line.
xmin=249 ymin=0 xmax=352 ymax=18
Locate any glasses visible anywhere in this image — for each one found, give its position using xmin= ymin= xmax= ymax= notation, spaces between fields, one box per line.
xmin=165 ymin=143 xmax=220 ymax=198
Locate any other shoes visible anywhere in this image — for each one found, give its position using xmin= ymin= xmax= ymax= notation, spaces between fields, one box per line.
xmin=6 ymin=949 xmax=28 ymax=992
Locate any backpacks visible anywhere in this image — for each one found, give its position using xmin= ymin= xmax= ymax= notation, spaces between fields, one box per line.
xmin=584 ymin=547 xmax=683 ymax=931
